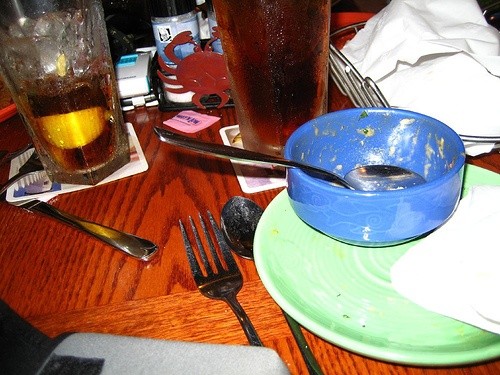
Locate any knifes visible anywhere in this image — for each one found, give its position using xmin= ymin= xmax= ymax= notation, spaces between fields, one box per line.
xmin=1 ymin=190 xmax=161 ymax=263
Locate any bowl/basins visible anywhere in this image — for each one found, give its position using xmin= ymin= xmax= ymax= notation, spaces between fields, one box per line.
xmin=283 ymin=107 xmax=466 ymax=249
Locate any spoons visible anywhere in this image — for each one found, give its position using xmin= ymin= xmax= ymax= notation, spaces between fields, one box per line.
xmin=152 ymin=126 xmax=426 ymax=192
xmin=220 ymin=195 xmax=324 ymax=375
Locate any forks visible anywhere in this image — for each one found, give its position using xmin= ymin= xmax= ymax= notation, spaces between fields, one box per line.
xmin=178 ymin=209 xmax=265 ymax=348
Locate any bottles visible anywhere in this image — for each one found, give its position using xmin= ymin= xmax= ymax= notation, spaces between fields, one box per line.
xmin=148 ymin=1 xmax=231 ymax=105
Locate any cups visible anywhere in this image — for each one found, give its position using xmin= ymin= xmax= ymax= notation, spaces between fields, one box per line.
xmin=0 ymin=0 xmax=130 ymax=186
xmin=212 ymin=0 xmax=332 ymax=163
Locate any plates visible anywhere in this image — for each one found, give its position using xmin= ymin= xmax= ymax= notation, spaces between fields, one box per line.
xmin=251 ymin=163 xmax=499 ymax=367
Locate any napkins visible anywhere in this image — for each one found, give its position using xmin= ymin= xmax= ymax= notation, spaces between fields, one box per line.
xmin=390 ymin=185 xmax=499 ymax=335
xmin=334 ymin=0 xmax=500 ymax=157
